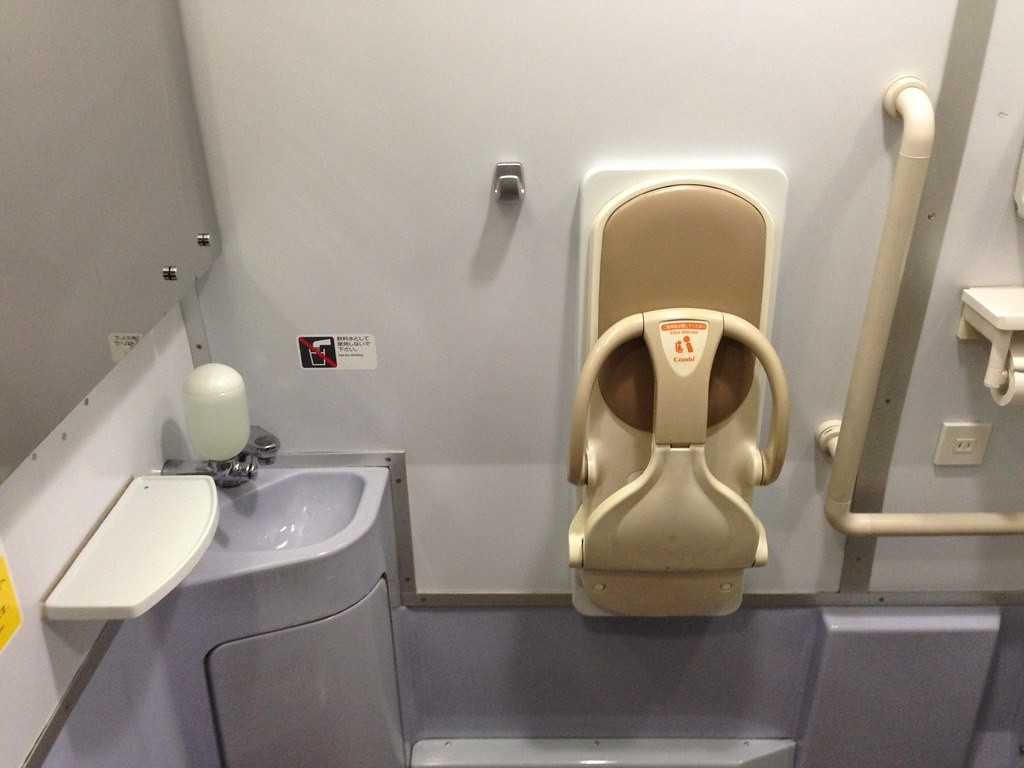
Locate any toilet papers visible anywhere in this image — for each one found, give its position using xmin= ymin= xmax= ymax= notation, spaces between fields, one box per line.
xmin=988 ymin=344 xmax=1024 ymax=409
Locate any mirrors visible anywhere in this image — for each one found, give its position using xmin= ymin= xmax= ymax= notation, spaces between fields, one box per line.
xmin=0 ymin=1 xmax=223 ymax=488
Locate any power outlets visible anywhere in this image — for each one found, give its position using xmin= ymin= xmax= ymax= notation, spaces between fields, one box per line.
xmin=932 ymin=419 xmax=994 ymax=467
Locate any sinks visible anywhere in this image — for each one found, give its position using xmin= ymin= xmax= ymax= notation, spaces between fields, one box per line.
xmin=205 ymin=468 xmax=368 ymax=554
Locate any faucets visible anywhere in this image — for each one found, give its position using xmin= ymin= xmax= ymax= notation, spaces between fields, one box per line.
xmin=160 ymin=424 xmax=283 ymax=490
